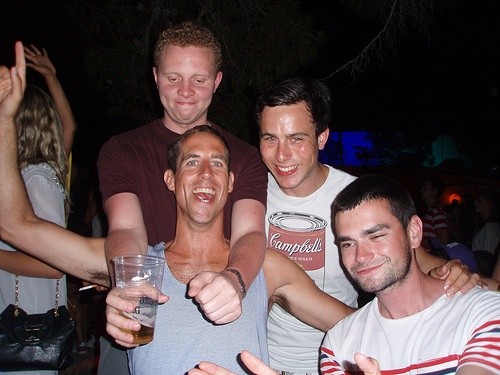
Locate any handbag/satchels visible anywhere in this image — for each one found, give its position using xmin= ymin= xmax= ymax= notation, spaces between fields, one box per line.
xmin=0 ymin=303 xmax=76 ymax=373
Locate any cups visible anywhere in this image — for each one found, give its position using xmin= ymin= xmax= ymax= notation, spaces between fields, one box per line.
xmin=112 ymin=254 xmax=165 ymax=345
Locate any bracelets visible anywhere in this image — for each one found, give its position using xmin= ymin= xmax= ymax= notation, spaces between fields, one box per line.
xmin=224 ymin=269 xmax=247 ymax=300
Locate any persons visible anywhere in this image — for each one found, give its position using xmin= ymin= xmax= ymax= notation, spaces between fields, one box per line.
xmin=0 ymin=86 xmax=69 ymax=375
xmin=186 ymin=174 xmax=500 ymax=375
xmin=68 ymin=167 xmax=96 ymax=354
xmin=255 ymin=76 xmax=488 ymax=375
xmin=95 ymin=24 xmax=269 ymax=375
xmin=23 ymin=44 xmax=77 ymax=228
xmin=0 ymin=41 xmax=488 ymax=375
xmin=409 ymin=175 xmax=499 ymax=292
